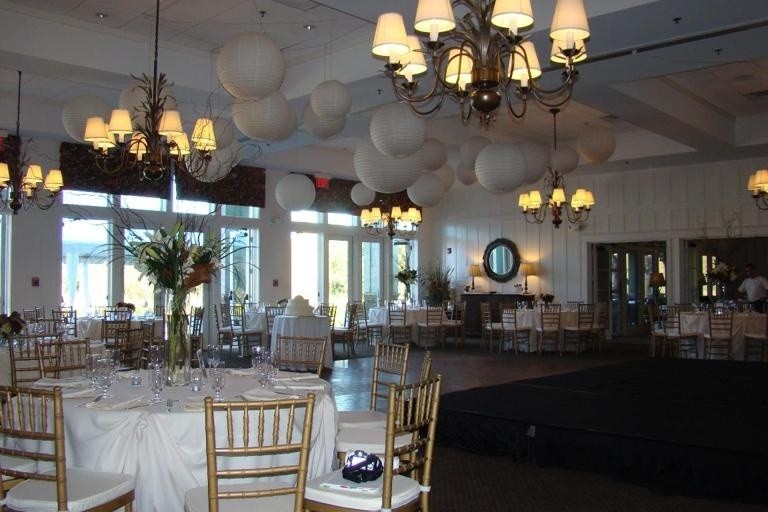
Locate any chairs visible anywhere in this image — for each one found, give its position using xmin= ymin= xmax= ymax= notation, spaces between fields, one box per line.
xmin=646 ymin=302 xmax=680 ymax=358
xmin=294 ymin=373 xmax=442 ymax=512
xmin=99 ymin=309 xmax=132 ymax=365
xmin=336 ymin=350 xmax=433 ymax=481
xmin=34 ymin=337 xmax=93 ymax=380
xmin=562 ymin=303 xmax=596 ymax=356
xmin=154 ymin=304 xmax=167 ymax=317
xmin=1 ymin=384 xmax=136 ymax=511
xmin=674 ymin=303 xmax=696 ymax=313
xmin=184 ymin=393 xmax=317 ymax=511
xmin=5 ymin=332 xmax=63 ymax=397
xmin=331 ymin=301 xmax=358 ymax=356
xmin=582 ymin=302 xmax=609 ymax=354
xmin=535 ymin=303 xmax=562 ymax=357
xmin=214 ymin=303 xmax=245 ymax=355
xmin=498 ymin=303 xmax=531 ymax=355
xmin=387 ymin=302 xmax=413 ymax=347
xmin=275 ymin=336 xmax=327 ymax=378
xmin=704 ymin=309 xmax=736 ymax=360
xmin=745 ymin=314 xmax=767 ymax=361
xmin=264 ymin=305 xmax=286 ymax=349
xmin=220 ymin=303 xmax=242 ymax=351
xmin=352 ymin=300 xmax=386 ymax=352
xmin=442 ymin=300 xmax=464 ymax=347
xmin=34 ymin=318 xmax=62 ymax=336
xmin=566 ymin=301 xmax=583 ymax=311
xmin=658 ymin=304 xmax=700 ymax=360
xmin=479 ymin=303 xmax=504 ymax=353
xmin=110 ymin=326 xmax=146 ymax=372
xmin=311 ymin=304 xmax=338 ymax=358
xmin=363 ymin=291 xmax=378 ymax=320
xmin=228 ymin=304 xmax=264 ymax=360
xmin=186 ymin=305 xmax=206 ymax=362
xmin=139 ymin=319 xmax=166 ymax=368
xmin=737 ymin=301 xmax=762 ymax=314
xmin=22 ymin=307 xmax=46 ymax=335
xmin=337 ymin=335 xmax=410 ymax=430
xmin=51 ymin=308 xmax=78 ymax=338
xmin=416 ymin=302 xmax=445 ymax=350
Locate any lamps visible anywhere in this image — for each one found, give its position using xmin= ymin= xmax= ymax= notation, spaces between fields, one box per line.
xmin=232 ymin=91 xmax=297 ymax=142
xmin=436 ymin=163 xmax=454 ymax=190
xmin=198 ymin=49 xmax=235 ymax=150
xmin=352 ymin=135 xmax=424 ymax=193
xmin=304 ymin=97 xmax=347 ymax=139
xmin=647 ymin=271 xmax=665 ymax=303
xmin=370 ymin=0 xmax=592 ymax=131
xmin=406 ymin=171 xmax=445 ymax=206
xmin=519 ymin=141 xmax=548 ymax=185
xmin=475 ymin=142 xmax=527 ymax=194
xmin=455 ymin=162 xmax=477 ymax=186
xmin=309 ymin=81 xmax=352 ymax=122
xmin=517 ymin=262 xmax=536 ymax=293
xmin=576 ymin=123 xmax=615 ymax=166
xmin=423 ymin=138 xmax=447 ymax=170
xmin=81 ymin=76 xmax=216 ymax=182
xmin=358 ymin=194 xmax=423 ymax=240
xmin=0 ymin=133 xmax=65 ymax=215
xmin=747 ymin=167 xmax=768 ymax=212
xmin=275 ymin=173 xmax=316 ymax=211
xmin=460 ymin=136 xmax=492 ymax=166
xmin=214 ymin=31 xmax=286 ymax=102
xmin=467 ymin=264 xmax=481 ymax=290
xmin=517 ymin=165 xmax=595 ymax=232
xmin=232 ymin=139 xmax=241 ymax=167
xmin=550 ymin=145 xmax=579 ymax=173
xmin=183 ymin=144 xmax=232 ymax=183
xmin=370 ymin=102 xmax=429 ymax=160
xmin=350 ymin=182 xmax=376 ymax=206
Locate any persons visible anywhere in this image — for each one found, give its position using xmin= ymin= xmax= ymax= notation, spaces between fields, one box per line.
xmin=733 ymin=263 xmax=767 ymax=313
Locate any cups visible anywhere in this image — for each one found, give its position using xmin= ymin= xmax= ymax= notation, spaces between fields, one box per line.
xmin=130 ymin=368 xmax=144 ymax=386
xmin=517 ymin=300 xmax=523 ymax=309
xmin=88 ymin=349 xmax=121 ymax=399
xmin=521 ymin=300 xmax=528 ymax=309
xmin=532 ymin=300 xmax=537 ymax=310
xmin=190 ymin=368 xmax=203 ymax=391
xmin=148 ymin=345 xmax=166 ymax=404
xmin=202 ymin=344 xmax=226 ymax=400
xmin=252 ymin=350 xmax=278 ymax=387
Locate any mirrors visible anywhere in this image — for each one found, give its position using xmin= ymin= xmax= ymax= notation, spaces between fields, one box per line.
xmin=483 ymin=238 xmax=521 ymax=282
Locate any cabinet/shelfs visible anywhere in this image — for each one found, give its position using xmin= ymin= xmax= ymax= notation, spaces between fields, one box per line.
xmin=460 ymin=292 xmax=535 ymax=339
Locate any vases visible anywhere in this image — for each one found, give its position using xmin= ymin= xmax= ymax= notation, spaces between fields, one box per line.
xmin=165 ymin=314 xmax=193 ymax=387
xmin=118 ymin=308 xmax=131 ymax=319
xmin=403 ymin=288 xmax=412 ymax=306
xmin=714 ymin=287 xmax=724 ymax=308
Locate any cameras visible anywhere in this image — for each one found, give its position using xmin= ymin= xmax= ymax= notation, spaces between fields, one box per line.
xmin=342 ymin=450 xmax=383 ymax=483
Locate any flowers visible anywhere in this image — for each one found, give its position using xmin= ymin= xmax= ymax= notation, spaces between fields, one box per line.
xmin=85 ymin=192 xmax=265 ymax=323
xmin=113 ymin=301 xmax=136 ymax=319
xmin=394 ymin=269 xmax=421 ymax=293
xmin=703 ymin=261 xmax=739 ymax=285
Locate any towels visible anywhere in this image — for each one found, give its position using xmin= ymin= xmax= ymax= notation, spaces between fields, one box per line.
xmin=96 ymin=390 xmax=144 ymax=410
xmin=232 ymin=366 xmax=257 ymax=376
xmin=241 ymin=385 xmax=300 ymax=401
xmin=275 ymin=380 xmax=325 ymax=390
xmin=183 ymin=394 xmax=213 ymax=410
xmin=275 ymin=371 xmax=318 ymax=380
xmin=36 ymin=377 xmax=94 ymax=395
